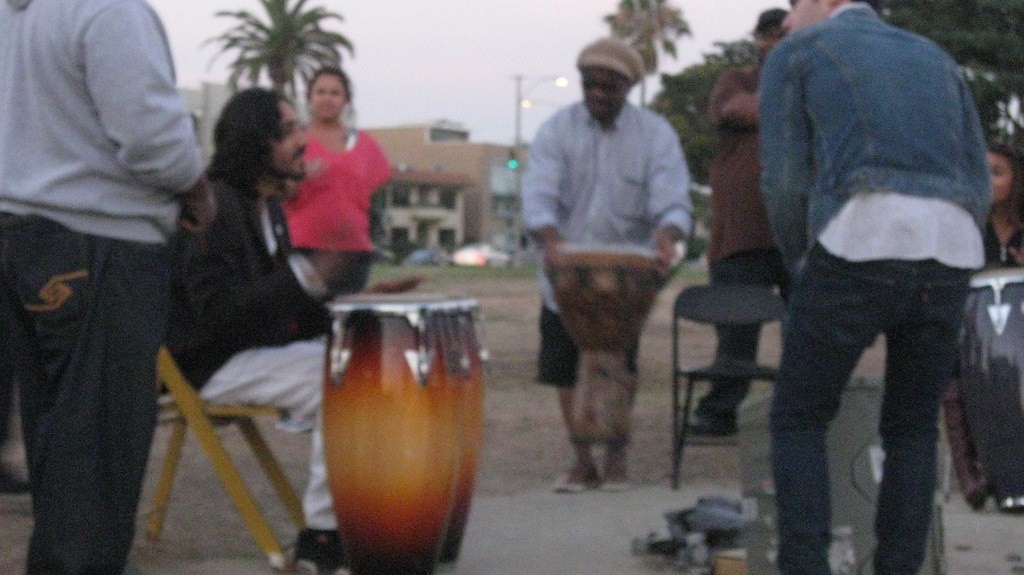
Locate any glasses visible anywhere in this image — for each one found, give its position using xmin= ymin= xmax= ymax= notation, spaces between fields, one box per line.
xmin=584 ymin=78 xmax=621 ymax=93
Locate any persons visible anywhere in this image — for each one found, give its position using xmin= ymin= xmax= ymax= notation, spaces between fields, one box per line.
xmin=519 ymin=37 xmax=693 ymax=493
xmin=682 ymin=8 xmax=789 ymax=437
xmin=161 ymin=87 xmax=351 ymax=575
xmin=756 ymin=0 xmax=992 ymax=575
xmin=0 ymin=0 xmax=219 ymax=575
xmin=278 ymin=67 xmax=395 ymax=297
xmin=982 ymin=141 xmax=1024 ymax=268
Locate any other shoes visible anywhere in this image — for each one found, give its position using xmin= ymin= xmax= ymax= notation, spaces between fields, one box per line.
xmin=685 ymin=412 xmax=737 ymax=437
xmin=604 ymin=457 xmax=629 ymax=493
xmin=293 ymin=530 xmax=347 ymax=573
xmin=555 ymin=455 xmax=596 ymax=493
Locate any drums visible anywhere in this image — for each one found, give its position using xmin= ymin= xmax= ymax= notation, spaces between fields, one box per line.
xmin=544 ymin=247 xmax=676 ymax=449
xmin=961 ymin=267 xmax=1023 ymax=514
xmin=323 ymin=295 xmax=490 ymax=574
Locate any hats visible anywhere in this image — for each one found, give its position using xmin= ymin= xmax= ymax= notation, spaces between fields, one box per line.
xmin=577 ymin=39 xmax=646 ymax=87
xmin=757 ymin=10 xmax=790 ymax=31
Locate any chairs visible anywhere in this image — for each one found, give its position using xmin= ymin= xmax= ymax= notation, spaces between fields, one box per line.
xmin=672 ymin=283 xmax=787 ymax=490
xmin=143 ymin=346 xmax=305 ymax=570
